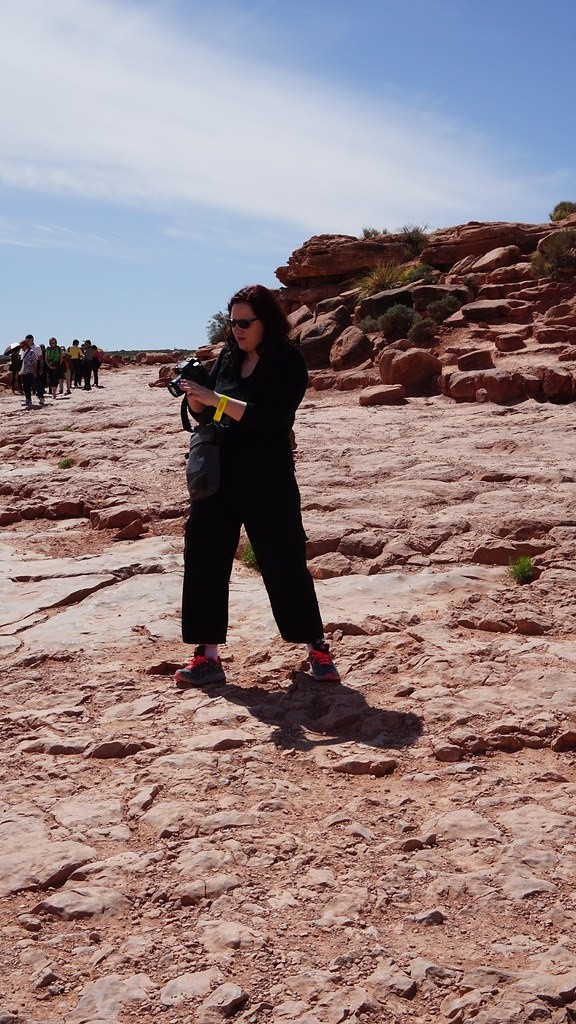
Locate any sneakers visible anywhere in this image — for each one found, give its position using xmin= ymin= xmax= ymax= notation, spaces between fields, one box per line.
xmin=175 ymin=645 xmax=225 ymax=685
xmin=308 ymin=642 xmax=341 ymax=681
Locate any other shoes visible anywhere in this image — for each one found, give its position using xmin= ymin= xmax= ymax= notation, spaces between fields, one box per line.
xmin=21 ymin=399 xmax=31 ymax=406
xmin=39 ymin=397 xmax=45 ymax=404
xmin=52 ymin=384 xmax=97 ymax=398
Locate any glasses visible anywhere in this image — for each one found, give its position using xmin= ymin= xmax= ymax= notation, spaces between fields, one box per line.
xmin=226 ymin=317 xmax=259 ymax=330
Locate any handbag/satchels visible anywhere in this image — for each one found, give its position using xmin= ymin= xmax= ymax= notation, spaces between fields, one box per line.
xmin=16 ymin=360 xmax=23 ymax=372
xmin=9 ymin=364 xmax=17 ymax=371
xmin=186 ymin=422 xmax=218 ymax=504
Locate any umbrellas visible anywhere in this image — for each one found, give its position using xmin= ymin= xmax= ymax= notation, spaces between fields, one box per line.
xmin=3 ymin=343 xmax=21 ymax=355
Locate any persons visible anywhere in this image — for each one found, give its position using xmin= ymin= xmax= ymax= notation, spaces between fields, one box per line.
xmin=11 ymin=348 xmax=23 ymax=394
xmin=67 ymin=339 xmax=101 ymax=391
xmin=171 ymin=285 xmax=341 ymax=687
xmin=39 ymin=338 xmax=71 ymax=399
xmin=19 ymin=335 xmax=45 ymax=406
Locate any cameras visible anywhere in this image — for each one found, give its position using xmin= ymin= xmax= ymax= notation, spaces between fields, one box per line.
xmin=167 ymin=358 xmax=211 ymax=398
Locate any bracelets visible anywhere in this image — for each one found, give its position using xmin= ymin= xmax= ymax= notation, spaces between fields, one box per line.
xmin=213 ymin=395 xmax=229 ymax=422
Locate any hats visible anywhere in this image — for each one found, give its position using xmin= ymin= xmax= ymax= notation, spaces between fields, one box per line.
xmin=83 ymin=340 xmax=91 ymax=345
xmin=60 ymin=346 xmax=65 ymax=350
